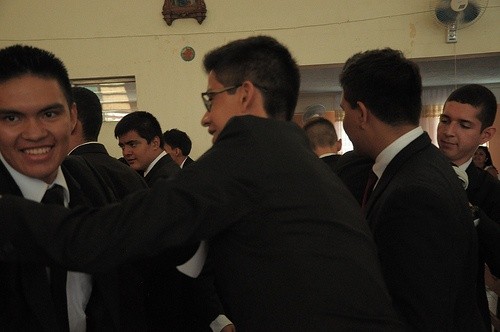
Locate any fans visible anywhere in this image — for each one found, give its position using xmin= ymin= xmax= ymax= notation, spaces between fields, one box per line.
xmin=430 ymin=0 xmax=489 ymax=44
xmin=301 ymin=104 xmax=329 ymax=124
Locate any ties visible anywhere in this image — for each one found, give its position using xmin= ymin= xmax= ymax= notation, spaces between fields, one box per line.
xmin=42 ymin=185 xmax=71 ymax=332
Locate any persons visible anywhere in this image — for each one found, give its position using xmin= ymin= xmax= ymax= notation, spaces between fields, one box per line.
xmin=473 ymin=146 xmax=498 ymax=180
xmin=303 ymin=117 xmax=342 ymax=176
xmin=0 ymin=34 xmax=412 ymax=332
xmin=0 ymin=44 xmax=237 ymax=332
xmin=437 ymin=85 xmax=500 ymax=332
xmin=115 ymin=111 xmax=194 ymax=185
xmin=335 ymin=47 xmax=488 ymax=332
xmin=61 ymin=87 xmax=147 ymax=208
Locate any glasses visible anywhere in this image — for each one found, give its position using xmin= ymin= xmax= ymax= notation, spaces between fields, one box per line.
xmin=201 ymin=85 xmax=267 ymax=111
